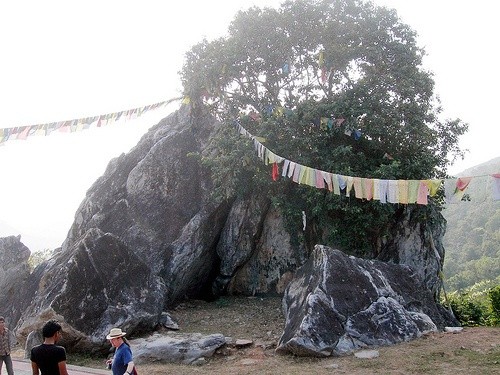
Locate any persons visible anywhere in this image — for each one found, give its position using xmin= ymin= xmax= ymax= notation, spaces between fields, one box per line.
xmin=30 ymin=319 xmax=69 ymax=375
xmin=105 ymin=328 xmax=135 ymax=375
xmin=0 ymin=316 xmax=14 ymax=375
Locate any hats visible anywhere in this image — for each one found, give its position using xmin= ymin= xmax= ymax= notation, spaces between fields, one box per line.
xmin=107 ymin=328 xmax=126 ymax=340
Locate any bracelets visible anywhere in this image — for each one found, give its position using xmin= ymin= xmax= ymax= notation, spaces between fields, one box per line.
xmin=126 ymin=371 xmax=131 ymax=375
